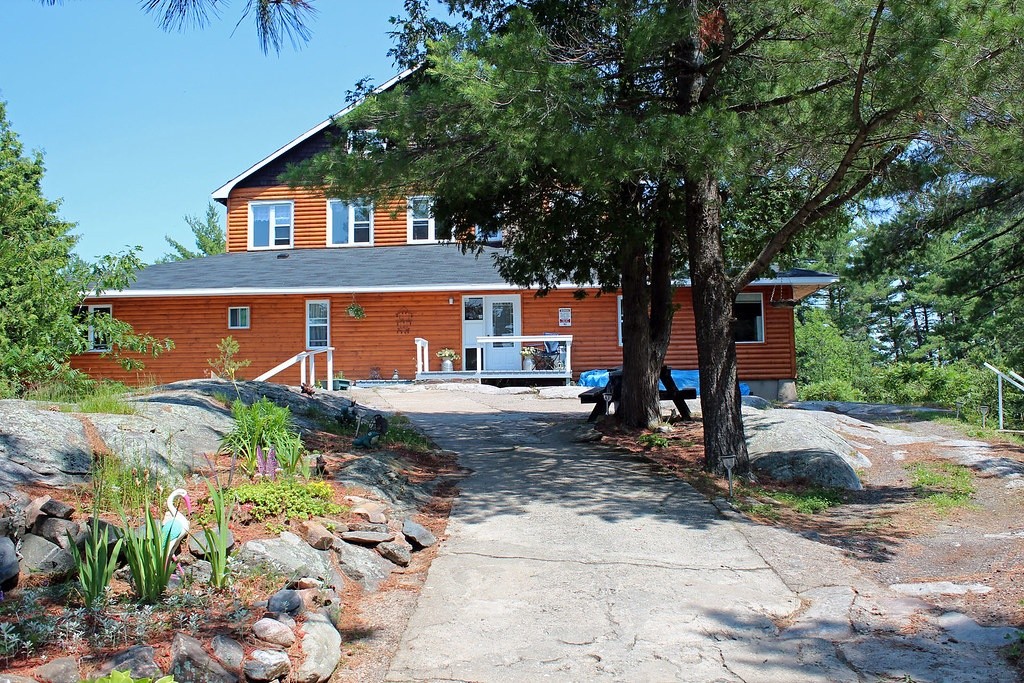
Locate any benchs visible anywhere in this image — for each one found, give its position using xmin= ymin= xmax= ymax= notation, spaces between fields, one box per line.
xmin=578 ymin=386 xmax=697 ymax=404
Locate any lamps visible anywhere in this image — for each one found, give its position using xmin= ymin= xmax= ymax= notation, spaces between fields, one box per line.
xmin=449 ymin=296 xmax=454 ymax=304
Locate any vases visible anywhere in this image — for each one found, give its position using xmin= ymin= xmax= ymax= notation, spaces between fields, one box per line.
xmin=442 ymin=358 xmax=453 ymax=371
xmin=522 ymin=355 xmax=533 ymax=370
xmin=348 ymin=309 xmax=355 ymax=317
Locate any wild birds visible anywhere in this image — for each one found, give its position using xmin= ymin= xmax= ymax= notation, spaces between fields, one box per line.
xmin=161 ymin=488 xmax=191 ymax=585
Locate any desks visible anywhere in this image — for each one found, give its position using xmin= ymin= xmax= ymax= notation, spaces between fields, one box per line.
xmin=587 ymin=362 xmax=691 ymax=424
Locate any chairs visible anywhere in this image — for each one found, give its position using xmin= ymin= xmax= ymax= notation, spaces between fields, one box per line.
xmin=528 ymin=332 xmax=559 ymax=370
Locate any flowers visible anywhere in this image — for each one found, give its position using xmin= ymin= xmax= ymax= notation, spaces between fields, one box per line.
xmin=520 ymin=346 xmax=537 ymax=357
xmin=347 ymin=304 xmax=366 ymax=319
xmin=435 ymin=348 xmax=460 ymax=361
